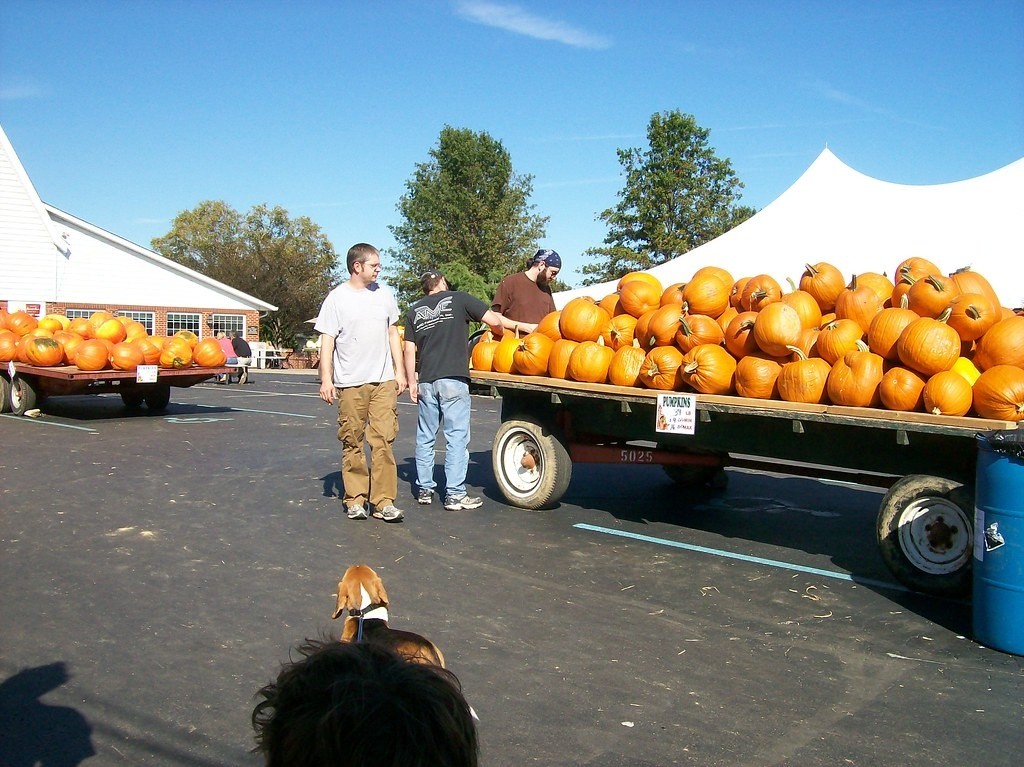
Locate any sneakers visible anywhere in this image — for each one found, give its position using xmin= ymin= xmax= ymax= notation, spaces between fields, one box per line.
xmin=445 ymin=494 xmax=483 ymax=511
xmin=418 ymin=488 xmax=432 ymax=504
xmin=373 ymin=504 xmax=404 ymax=520
xmin=347 ymin=503 xmax=367 ymax=519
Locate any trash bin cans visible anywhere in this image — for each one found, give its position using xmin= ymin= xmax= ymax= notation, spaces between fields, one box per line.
xmin=970 ymin=426 xmax=1024 ymax=655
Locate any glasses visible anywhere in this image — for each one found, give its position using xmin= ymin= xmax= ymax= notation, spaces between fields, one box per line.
xmin=548 ymin=267 xmax=557 ymax=275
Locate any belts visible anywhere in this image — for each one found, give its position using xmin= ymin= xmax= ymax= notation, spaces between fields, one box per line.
xmin=239 ymin=355 xmax=251 ymax=358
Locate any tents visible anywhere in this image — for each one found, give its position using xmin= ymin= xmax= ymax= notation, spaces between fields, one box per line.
xmin=550 ymin=146 xmax=1024 ymax=306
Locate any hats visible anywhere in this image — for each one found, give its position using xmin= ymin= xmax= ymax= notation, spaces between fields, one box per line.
xmin=420 ymin=269 xmax=451 ymax=289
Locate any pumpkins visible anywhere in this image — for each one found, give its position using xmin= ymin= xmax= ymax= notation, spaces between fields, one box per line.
xmin=0 ymin=310 xmax=227 ymax=371
xmin=472 ymin=257 xmax=1024 ymax=426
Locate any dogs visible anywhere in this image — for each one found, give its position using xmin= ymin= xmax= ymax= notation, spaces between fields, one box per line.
xmin=330 ymin=563 xmax=480 ymax=725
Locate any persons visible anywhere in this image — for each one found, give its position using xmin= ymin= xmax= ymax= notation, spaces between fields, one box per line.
xmin=313 ymin=243 xmax=408 ymax=522
xmin=251 ymin=637 xmax=479 ymax=767
xmin=403 ymin=269 xmax=505 ymax=511
xmin=491 ymin=249 xmax=561 ymax=338
xmin=216 ymin=331 xmax=252 ymax=385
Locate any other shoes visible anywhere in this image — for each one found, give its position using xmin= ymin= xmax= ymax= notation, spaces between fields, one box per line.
xmin=220 ymin=377 xmax=227 ymax=382
xmin=239 ymin=372 xmax=247 ymax=384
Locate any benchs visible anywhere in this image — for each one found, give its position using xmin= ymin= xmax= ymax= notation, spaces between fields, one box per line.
xmin=224 ymin=364 xmax=249 ymax=384
